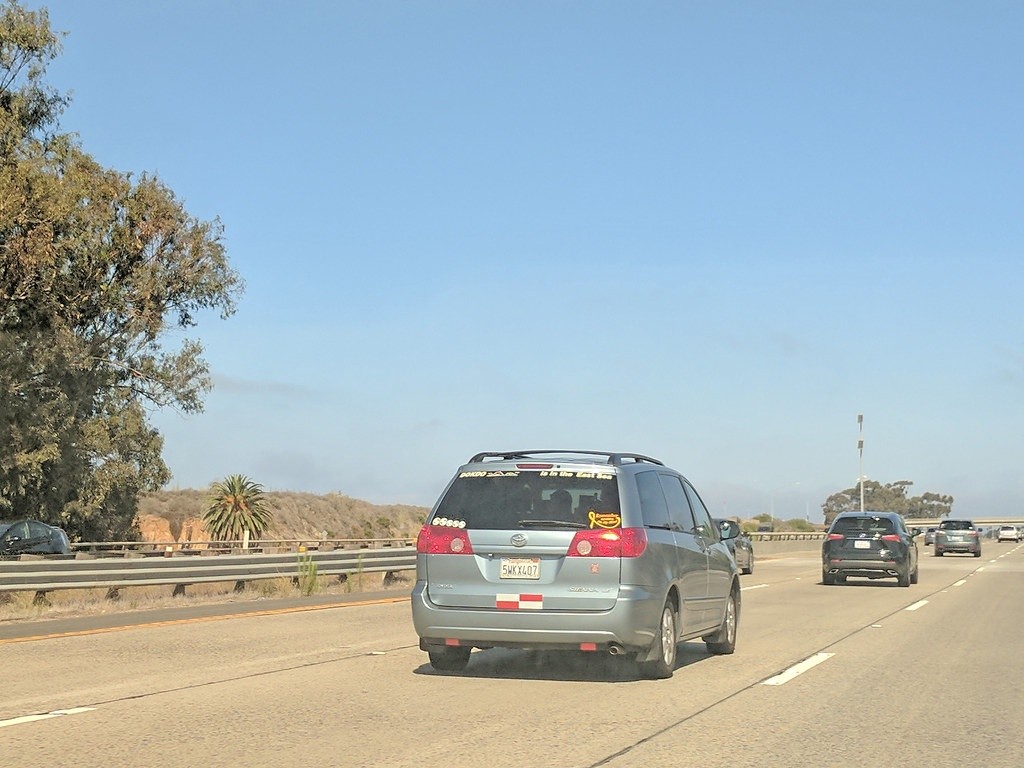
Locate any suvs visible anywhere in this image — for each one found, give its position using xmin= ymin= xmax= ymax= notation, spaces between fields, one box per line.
xmin=411 ymin=451 xmax=752 ymax=679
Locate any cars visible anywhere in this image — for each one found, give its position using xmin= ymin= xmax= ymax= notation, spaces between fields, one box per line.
xmin=756 ymin=522 xmax=773 ymax=532
xmin=0 ymin=517 xmax=71 ymax=555
xmin=712 ymin=518 xmax=754 ymax=575
xmin=925 ymin=527 xmax=937 ymax=545
xmin=934 ymin=520 xmax=983 ymax=557
xmin=822 ymin=511 xmax=924 ymax=587
xmin=997 ymin=525 xmax=1019 ymax=543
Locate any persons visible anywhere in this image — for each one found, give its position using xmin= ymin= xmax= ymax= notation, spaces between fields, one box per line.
xmin=549 ymin=490 xmax=572 ymax=515
